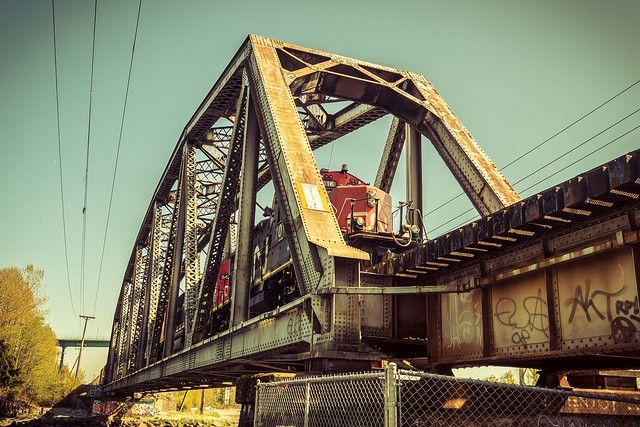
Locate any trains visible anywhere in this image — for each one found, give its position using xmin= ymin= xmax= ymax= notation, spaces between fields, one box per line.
xmin=93 ymin=164 xmax=425 ymax=381
